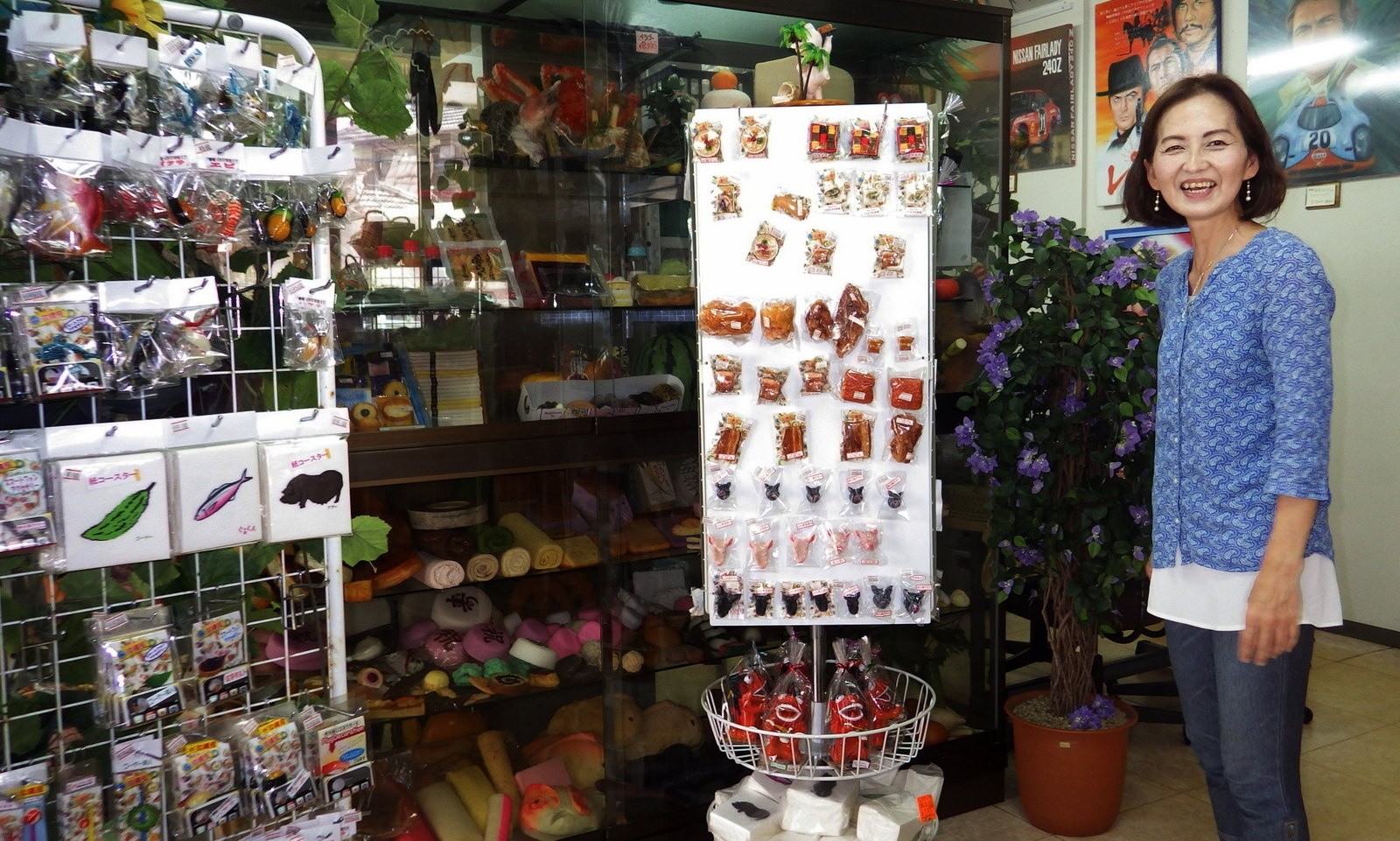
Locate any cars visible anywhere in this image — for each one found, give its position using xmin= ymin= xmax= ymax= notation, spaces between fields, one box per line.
xmin=971 ymin=90 xmax=1064 ymax=162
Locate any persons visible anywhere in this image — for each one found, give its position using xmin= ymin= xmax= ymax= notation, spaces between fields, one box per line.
xmin=1120 ymin=73 xmax=1344 ymax=841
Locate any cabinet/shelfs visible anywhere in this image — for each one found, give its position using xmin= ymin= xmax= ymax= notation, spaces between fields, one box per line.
xmin=328 ymin=0 xmax=1016 ymax=841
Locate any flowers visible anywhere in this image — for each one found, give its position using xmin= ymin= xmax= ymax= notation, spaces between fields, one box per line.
xmin=955 ymin=210 xmax=1171 ymax=732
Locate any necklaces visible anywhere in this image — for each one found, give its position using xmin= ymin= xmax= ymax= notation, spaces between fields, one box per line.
xmin=1181 ymin=222 xmax=1241 ymax=322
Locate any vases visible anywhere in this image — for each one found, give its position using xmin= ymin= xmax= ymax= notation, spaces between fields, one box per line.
xmin=1004 ymin=690 xmax=1138 ymax=836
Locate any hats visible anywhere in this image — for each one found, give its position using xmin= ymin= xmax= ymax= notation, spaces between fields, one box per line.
xmin=1096 ymin=55 xmax=1146 ymax=97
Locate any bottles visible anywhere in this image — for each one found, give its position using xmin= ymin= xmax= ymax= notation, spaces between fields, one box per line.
xmin=376 ymin=245 xmax=394 ymax=266
xmin=403 ymin=240 xmax=420 ymax=266
xmin=626 ymin=233 xmax=648 ymax=282
xmin=426 ymin=245 xmax=442 ymax=280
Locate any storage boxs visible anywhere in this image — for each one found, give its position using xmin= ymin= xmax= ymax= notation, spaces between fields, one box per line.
xmin=517 ymin=373 xmax=684 ymax=425
